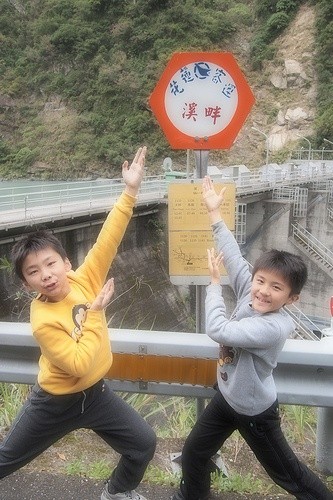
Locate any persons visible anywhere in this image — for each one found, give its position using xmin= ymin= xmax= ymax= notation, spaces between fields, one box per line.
xmin=171 ymin=173 xmax=332 ymax=500
xmin=0 ymin=145 xmax=158 ymax=500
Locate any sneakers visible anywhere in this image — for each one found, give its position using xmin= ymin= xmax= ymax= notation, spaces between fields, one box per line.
xmin=100 ymin=481 xmax=149 ymax=500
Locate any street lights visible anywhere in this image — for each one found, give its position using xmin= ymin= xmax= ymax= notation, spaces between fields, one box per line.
xmin=251 ymin=126 xmax=270 ymax=173
xmin=296 ymin=134 xmax=311 ymax=159
